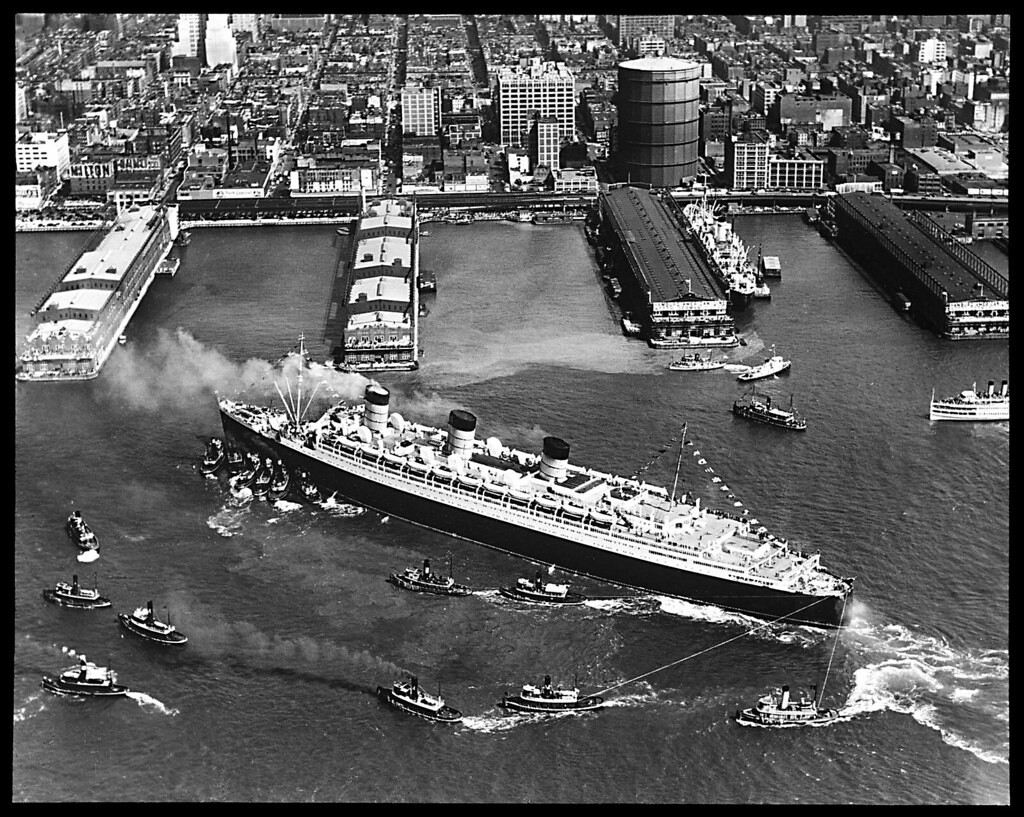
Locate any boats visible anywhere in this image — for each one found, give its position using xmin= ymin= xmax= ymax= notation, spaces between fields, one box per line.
xmin=376 ymin=668 xmax=463 ymax=724
xmin=668 ymin=348 xmax=726 ymax=374
xmin=735 ymin=682 xmax=838 ymax=726
xmin=733 ymin=380 xmax=805 ymax=432
xmin=500 ymin=573 xmax=589 ymax=607
xmin=42 ymin=573 xmax=113 ymax=608
xmin=67 ymin=511 xmax=101 ymax=554
xmin=926 ymin=380 xmax=1009 ymax=422
xmin=200 ymin=435 xmax=321 ymax=508
xmin=739 ymin=344 xmax=796 ymax=380
xmin=389 ymin=558 xmax=474 ymax=599
xmin=500 ymin=675 xmax=605 ymax=711
xmin=116 ymin=601 xmax=189 ymax=644
xmin=682 ymin=203 xmax=756 ymax=304
xmin=39 ymin=661 xmax=132 ymax=698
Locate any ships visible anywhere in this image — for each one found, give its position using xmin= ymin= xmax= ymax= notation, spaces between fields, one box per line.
xmin=212 ymin=330 xmax=853 ymax=631
